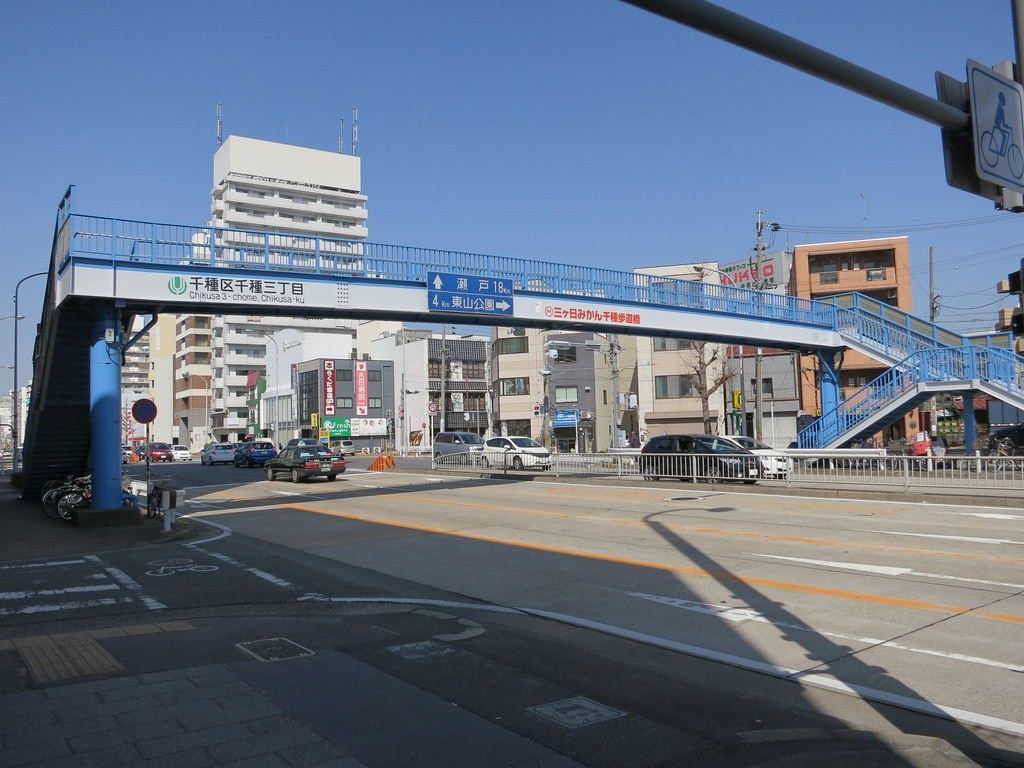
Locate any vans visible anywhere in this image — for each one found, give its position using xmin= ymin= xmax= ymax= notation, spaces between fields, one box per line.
xmin=329 ymin=440 xmax=355 ymax=456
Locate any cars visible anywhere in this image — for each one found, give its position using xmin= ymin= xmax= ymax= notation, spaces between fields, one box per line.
xmin=639 ymin=434 xmax=764 ymax=484
xmin=480 ymin=436 xmax=552 ymax=470
xmin=121 ymin=446 xmax=132 ymax=457
xmin=148 ymin=442 xmax=174 ymax=462
xmin=712 ymin=436 xmax=792 ymax=479
xmin=433 ymin=432 xmax=485 ymax=465
xmin=171 ymin=445 xmax=192 ymax=461
xmin=200 ymin=443 xmax=243 ymax=466
xmin=121 ymin=448 xmax=128 ymax=464
xmin=133 ymin=446 xmax=146 ymax=461
xmin=234 ymin=441 xmax=277 ymax=468
xmin=264 ymin=445 xmax=346 ymax=483
xmin=278 ymin=439 xmax=323 ymax=449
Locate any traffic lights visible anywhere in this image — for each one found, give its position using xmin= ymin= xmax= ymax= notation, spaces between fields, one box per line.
xmin=534 ymin=411 xmax=539 ymax=416
xmin=1011 ymin=312 xmax=1024 ymax=336
xmin=1008 ymin=270 xmax=1023 ymax=296
xmin=534 ymin=406 xmax=539 ymax=410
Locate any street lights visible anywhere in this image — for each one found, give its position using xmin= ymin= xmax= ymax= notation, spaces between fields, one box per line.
xmin=693 ymin=265 xmax=749 ymax=448
xmin=245 ymin=329 xmax=279 ymax=453
xmin=190 ymin=373 xmax=208 ymax=443
xmin=134 ymin=388 xmax=154 ymax=442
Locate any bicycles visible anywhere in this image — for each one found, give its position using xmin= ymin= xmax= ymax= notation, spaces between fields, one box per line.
xmin=148 ymin=473 xmax=173 ymax=521
xmin=987 ymin=438 xmax=1024 ymax=473
xmin=888 ymin=438 xmax=927 ymax=471
xmin=40 ymin=475 xmax=92 ymax=520
